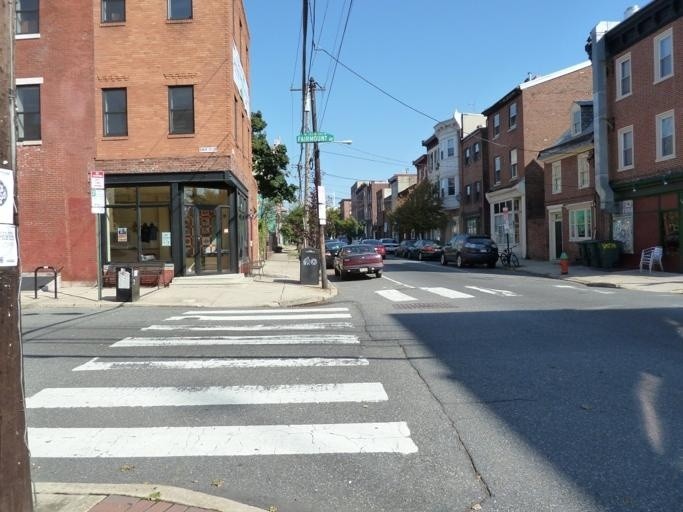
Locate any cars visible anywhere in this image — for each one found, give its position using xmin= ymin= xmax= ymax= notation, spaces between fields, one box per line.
xmin=325 ymin=234 xmax=499 ymax=281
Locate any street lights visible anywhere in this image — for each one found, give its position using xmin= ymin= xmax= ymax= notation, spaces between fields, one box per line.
xmin=305 ymin=140 xmax=352 ymax=245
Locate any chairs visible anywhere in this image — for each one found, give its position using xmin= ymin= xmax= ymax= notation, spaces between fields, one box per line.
xmin=639 ymin=245 xmax=665 ymax=272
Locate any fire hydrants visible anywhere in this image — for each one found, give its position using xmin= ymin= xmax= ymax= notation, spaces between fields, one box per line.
xmin=559 ymin=252 xmax=569 ymax=275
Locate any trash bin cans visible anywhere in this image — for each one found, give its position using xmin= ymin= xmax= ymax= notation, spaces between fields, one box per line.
xmin=299 ymin=248 xmax=321 ymax=285
xmin=115 ymin=266 xmax=140 ymax=302
xmin=576 ymin=240 xmax=624 ymax=272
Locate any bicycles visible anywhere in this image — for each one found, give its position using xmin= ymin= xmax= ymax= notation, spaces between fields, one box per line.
xmin=500 ymin=245 xmax=521 ymax=267
